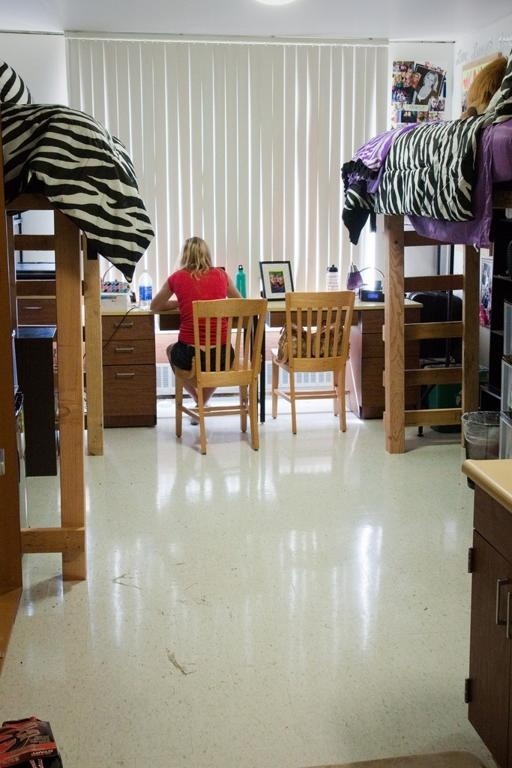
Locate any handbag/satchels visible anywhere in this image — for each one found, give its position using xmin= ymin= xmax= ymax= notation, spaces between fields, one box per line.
xmin=273 ymin=320 xmax=349 ymax=365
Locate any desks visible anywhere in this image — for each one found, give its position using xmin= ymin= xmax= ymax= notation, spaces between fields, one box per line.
xmin=100 ymin=296 xmax=425 ymax=428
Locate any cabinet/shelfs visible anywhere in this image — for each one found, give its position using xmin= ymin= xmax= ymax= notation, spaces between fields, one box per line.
xmin=464 ymin=485 xmax=512 ymax=768
xmin=18 ymin=276 xmax=58 ymax=397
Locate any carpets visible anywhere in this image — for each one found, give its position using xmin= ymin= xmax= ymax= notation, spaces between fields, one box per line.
xmin=304 ymin=751 xmax=486 ymax=767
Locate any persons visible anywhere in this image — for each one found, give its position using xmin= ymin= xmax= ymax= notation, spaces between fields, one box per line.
xmin=151 ymin=237 xmax=244 ymax=425
xmin=392 ymin=64 xmax=444 ymax=122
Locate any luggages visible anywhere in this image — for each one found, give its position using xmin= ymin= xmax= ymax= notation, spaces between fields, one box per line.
xmin=409 ymin=290 xmax=462 ymax=409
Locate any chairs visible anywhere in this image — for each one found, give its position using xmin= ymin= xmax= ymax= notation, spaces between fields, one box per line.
xmin=174 ymin=298 xmax=268 ymax=455
xmin=271 ymin=291 xmax=357 ymax=436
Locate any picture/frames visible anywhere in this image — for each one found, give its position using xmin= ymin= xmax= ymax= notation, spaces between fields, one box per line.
xmin=259 ymin=261 xmax=294 ymax=301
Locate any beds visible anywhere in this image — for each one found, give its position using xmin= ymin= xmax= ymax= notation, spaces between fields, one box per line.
xmin=0 ymin=58 xmax=155 ymax=580
xmin=340 ymin=117 xmax=512 ymax=453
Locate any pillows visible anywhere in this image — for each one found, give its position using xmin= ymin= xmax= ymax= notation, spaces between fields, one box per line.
xmin=491 ymin=48 xmax=512 ymax=126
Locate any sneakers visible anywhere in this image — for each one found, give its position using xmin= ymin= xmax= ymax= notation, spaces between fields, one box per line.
xmin=191 ymin=405 xmax=199 ymax=425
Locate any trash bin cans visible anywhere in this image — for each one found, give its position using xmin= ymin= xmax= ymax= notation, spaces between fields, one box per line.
xmin=461 ymin=410 xmax=501 ymax=488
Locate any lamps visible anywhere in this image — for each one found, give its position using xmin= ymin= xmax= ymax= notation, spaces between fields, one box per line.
xmin=347 ymin=261 xmax=385 ymax=290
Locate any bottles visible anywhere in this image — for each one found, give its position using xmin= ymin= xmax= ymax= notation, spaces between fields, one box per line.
xmin=139 ymin=268 xmax=153 ymax=310
xmin=326 ymin=264 xmax=339 ymax=290
xmin=236 ymin=265 xmax=247 ymax=297
